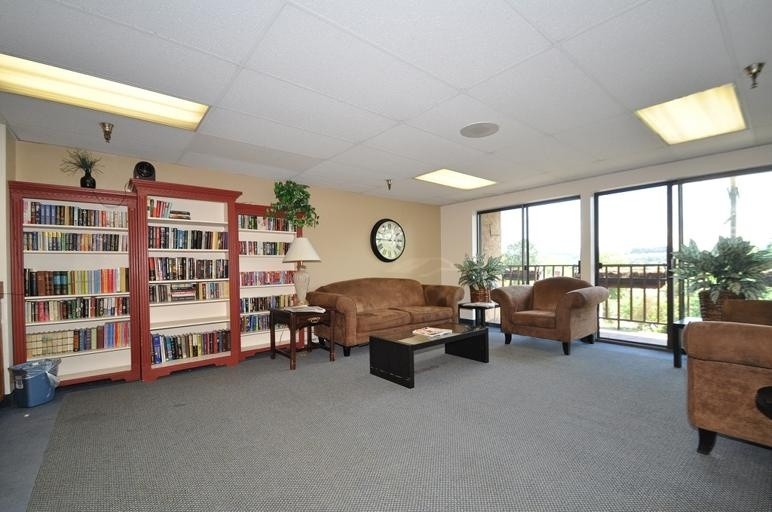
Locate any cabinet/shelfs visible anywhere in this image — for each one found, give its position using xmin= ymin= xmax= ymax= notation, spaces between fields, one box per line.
xmin=232 ymin=202 xmax=308 ymax=363
xmin=123 ymin=177 xmax=244 ymax=382
xmin=7 ymin=176 xmax=140 ymax=392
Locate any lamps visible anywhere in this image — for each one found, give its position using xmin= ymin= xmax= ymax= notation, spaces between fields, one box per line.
xmin=279 ymin=236 xmax=323 ymax=307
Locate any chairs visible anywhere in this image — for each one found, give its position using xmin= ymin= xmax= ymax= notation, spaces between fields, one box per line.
xmin=679 ymin=298 xmax=771 ymax=458
xmin=489 ymin=275 xmax=610 ymax=356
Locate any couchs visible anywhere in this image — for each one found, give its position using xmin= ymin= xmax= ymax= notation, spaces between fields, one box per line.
xmin=305 ymin=276 xmax=465 ymax=358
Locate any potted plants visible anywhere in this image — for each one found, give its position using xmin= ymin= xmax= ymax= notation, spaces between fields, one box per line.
xmin=263 ymin=179 xmax=320 ymax=231
xmin=452 ymin=249 xmax=510 ymax=303
xmin=662 ymin=232 xmax=772 ymax=322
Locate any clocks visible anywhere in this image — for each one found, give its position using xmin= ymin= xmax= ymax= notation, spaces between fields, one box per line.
xmin=369 ymin=218 xmax=406 ymax=262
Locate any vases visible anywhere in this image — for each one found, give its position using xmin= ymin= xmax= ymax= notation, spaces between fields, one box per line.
xmin=80 ymin=172 xmax=96 ymax=189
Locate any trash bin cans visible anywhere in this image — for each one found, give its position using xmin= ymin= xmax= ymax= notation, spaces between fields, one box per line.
xmin=7 ymin=358 xmax=61 ymax=408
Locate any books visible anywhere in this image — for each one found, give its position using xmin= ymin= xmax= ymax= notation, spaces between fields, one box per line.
xmin=24 ymin=232 xmax=129 ymax=251
xmin=240 ymin=270 xmax=295 ymax=286
xmin=240 ymin=294 xmax=297 ymax=332
xmin=25 ymin=296 xmax=130 ymax=323
xmin=24 ymin=200 xmax=128 ymax=228
xmin=147 ymin=226 xmax=227 ymax=249
xmin=147 ymin=200 xmax=191 ymax=220
xmin=148 ymin=257 xmax=228 ymax=280
xmin=150 ymin=329 xmax=230 ymax=364
xmin=24 ymin=268 xmax=130 ymax=297
xmin=412 ymin=327 xmax=453 ymax=337
xmin=150 ymin=282 xmax=230 ymax=303
xmin=26 ymin=321 xmax=131 ymax=359
xmin=239 ymin=241 xmax=290 ymax=255
xmin=238 ymin=215 xmax=297 ymax=232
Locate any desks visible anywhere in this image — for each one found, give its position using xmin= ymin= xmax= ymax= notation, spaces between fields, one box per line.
xmin=266 ymin=305 xmax=338 ymax=370
xmin=671 ymin=315 xmax=705 ymax=369
xmin=456 ymin=302 xmax=503 ymax=333
